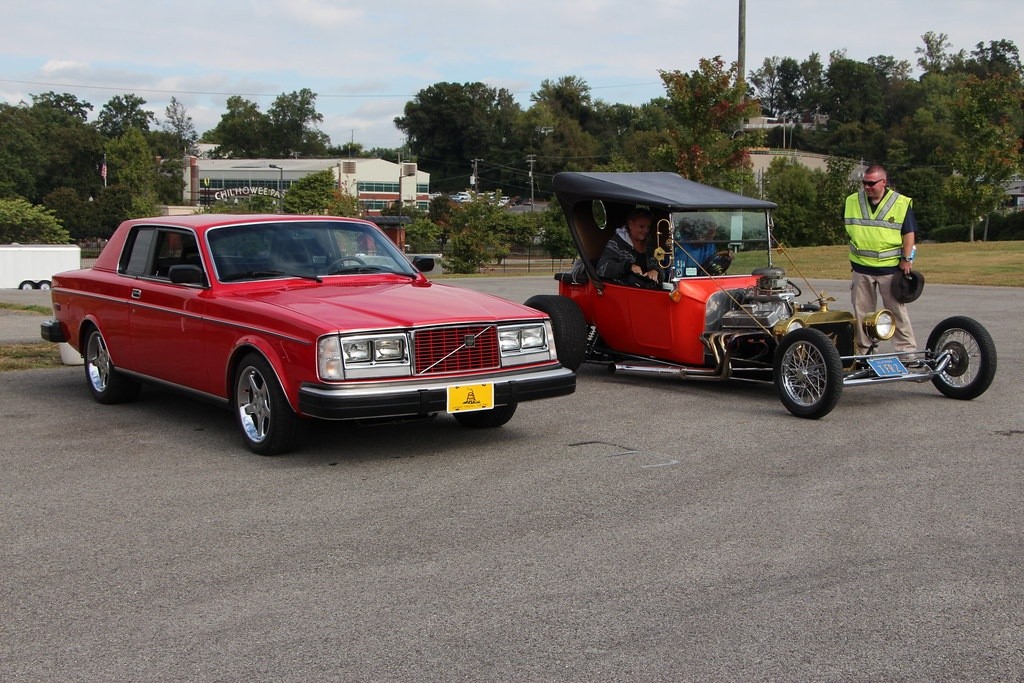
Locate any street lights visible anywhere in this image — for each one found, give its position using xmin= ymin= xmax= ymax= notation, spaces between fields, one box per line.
xmin=269 ymin=164 xmax=284 ymax=214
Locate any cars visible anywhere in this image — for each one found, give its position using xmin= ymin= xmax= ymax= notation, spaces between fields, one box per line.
xmin=41 ymin=214 xmax=576 ymax=457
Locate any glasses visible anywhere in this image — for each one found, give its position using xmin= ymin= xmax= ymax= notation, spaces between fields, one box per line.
xmin=861 ymin=178 xmax=883 ymax=186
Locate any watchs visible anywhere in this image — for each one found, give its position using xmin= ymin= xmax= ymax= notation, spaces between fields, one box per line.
xmin=903 ymin=256 xmax=912 ymax=262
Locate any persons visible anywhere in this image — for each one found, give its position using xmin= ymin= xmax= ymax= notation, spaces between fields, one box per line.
xmin=667 ymin=215 xmax=717 ymax=278
xmin=843 ymin=165 xmax=923 ymax=370
xmin=595 ymin=207 xmax=666 ymax=286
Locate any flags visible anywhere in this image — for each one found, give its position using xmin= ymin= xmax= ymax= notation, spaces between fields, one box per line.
xmin=101 ymin=159 xmax=107 ymax=179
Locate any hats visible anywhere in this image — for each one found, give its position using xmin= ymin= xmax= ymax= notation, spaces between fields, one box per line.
xmin=890 ymin=268 xmax=924 ymax=303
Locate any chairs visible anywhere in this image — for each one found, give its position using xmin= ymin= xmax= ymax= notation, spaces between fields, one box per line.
xmin=182 ymin=235 xmax=323 ymax=282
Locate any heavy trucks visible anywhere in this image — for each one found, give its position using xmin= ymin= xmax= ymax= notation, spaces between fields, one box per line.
xmin=0 ymin=244 xmax=81 ymax=289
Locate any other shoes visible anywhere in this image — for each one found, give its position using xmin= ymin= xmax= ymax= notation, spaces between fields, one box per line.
xmin=856 ymin=360 xmax=869 ymax=369
xmin=901 ymin=361 xmax=920 ymax=368
